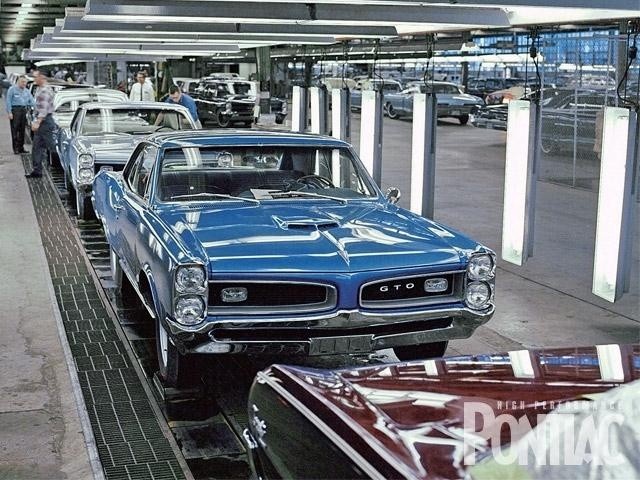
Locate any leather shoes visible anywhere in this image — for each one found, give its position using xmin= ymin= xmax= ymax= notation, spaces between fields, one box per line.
xmin=15 ymin=151 xmax=19 ymax=154
xmin=20 ymin=151 xmax=29 ymax=153
xmin=25 ymin=172 xmax=42 ymax=178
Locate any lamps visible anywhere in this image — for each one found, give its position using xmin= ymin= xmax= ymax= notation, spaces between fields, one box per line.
xmin=19 ymin=1 xmax=512 ymax=63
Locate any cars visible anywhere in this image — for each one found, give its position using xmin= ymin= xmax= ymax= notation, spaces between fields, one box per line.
xmin=172 ymin=72 xmax=288 ymax=128
xmin=244 ymin=344 xmax=640 ymax=479
xmin=468 ymin=73 xmax=630 ymax=156
xmin=319 ymin=70 xmax=469 ymax=126
xmin=27 ymin=76 xmax=197 ymax=218
xmin=92 ymin=130 xmax=498 ymax=387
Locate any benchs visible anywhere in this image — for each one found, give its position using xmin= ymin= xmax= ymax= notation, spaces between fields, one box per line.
xmin=161 ymin=170 xmax=306 ymax=201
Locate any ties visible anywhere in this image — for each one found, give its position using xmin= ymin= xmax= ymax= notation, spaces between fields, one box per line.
xmin=140 ymin=84 xmax=143 ymax=101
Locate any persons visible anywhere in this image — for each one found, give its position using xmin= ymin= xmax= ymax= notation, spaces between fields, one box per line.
xmin=155 ymin=85 xmax=208 ymax=130
xmin=6 ymin=74 xmax=35 ymax=154
xmin=131 ymin=68 xmax=155 ymax=125
xmin=25 ymin=70 xmax=57 ymax=178
